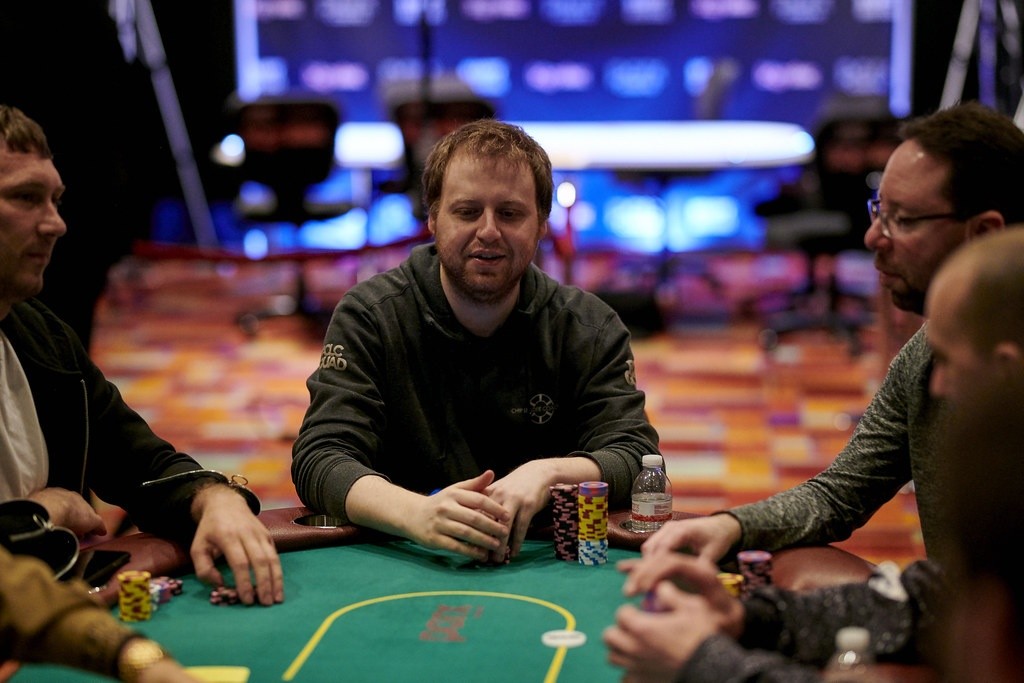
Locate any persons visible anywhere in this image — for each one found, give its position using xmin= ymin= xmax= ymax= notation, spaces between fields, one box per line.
xmin=640 ymin=102 xmax=1024 ymax=559
xmin=292 ymin=120 xmax=665 ymax=560
xmin=0 ymin=105 xmax=285 ymax=683
xmin=606 ymin=229 xmax=1024 ymax=683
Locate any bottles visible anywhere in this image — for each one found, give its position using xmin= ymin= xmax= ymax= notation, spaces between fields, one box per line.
xmin=631 ymin=455 xmax=672 ymax=533
xmin=823 ymin=627 xmax=890 ymax=683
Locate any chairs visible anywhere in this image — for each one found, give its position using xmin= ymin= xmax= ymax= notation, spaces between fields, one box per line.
xmin=616 ymin=74 xmax=729 ymax=322
xmin=749 ymin=111 xmax=905 ymax=358
xmin=371 ymin=94 xmax=495 ymax=217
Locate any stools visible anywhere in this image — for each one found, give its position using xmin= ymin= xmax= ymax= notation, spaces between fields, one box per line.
xmin=234 ymin=98 xmax=355 ymax=335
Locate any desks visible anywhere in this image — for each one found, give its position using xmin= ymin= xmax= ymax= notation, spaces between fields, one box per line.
xmin=0 ymin=505 xmax=939 ymax=683
xmin=209 ymin=114 xmax=815 ymax=336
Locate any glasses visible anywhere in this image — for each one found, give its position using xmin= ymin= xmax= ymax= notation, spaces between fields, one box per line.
xmin=867 ymin=196 xmax=973 ymax=236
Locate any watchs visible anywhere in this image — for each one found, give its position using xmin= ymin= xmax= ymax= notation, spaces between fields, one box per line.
xmin=122 ymin=639 xmax=171 ymax=682
